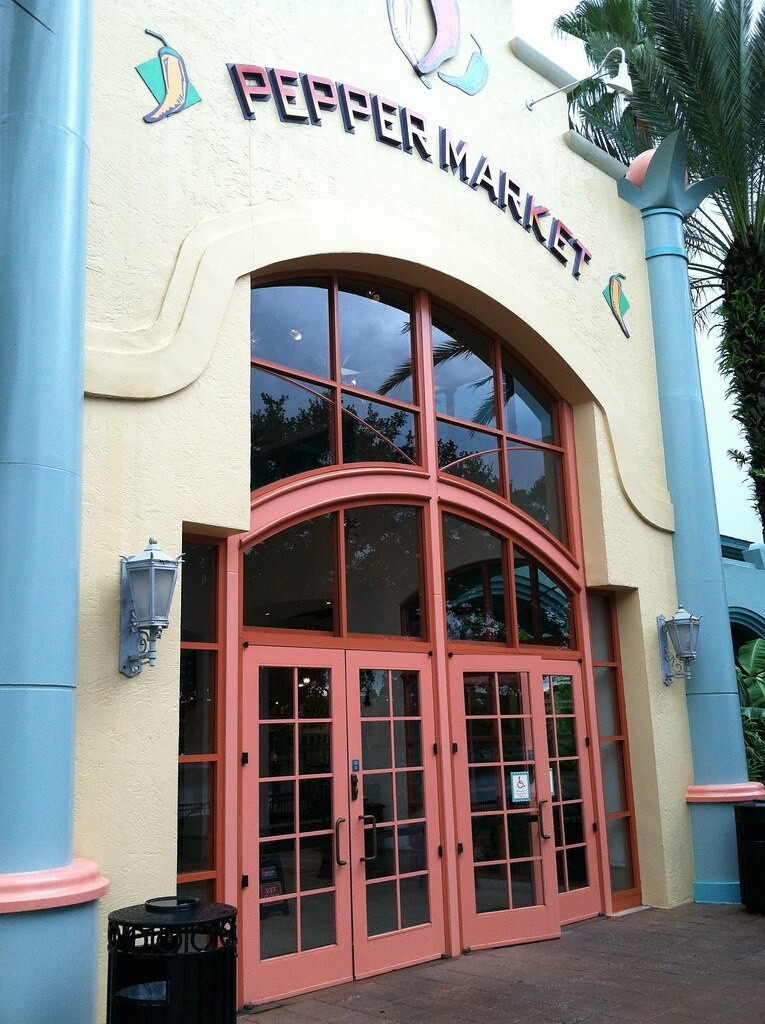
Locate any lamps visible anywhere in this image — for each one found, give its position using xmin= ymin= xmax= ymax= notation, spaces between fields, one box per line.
xmin=119 ymin=536 xmax=185 ymax=678
xmin=525 ymin=46 xmax=633 ymax=111
xmin=657 ymin=604 xmax=704 ymax=685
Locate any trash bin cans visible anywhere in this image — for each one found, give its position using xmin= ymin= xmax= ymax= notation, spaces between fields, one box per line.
xmin=734 ymin=799 xmax=765 ymax=915
xmin=107 ymin=896 xmax=237 ymax=1024
